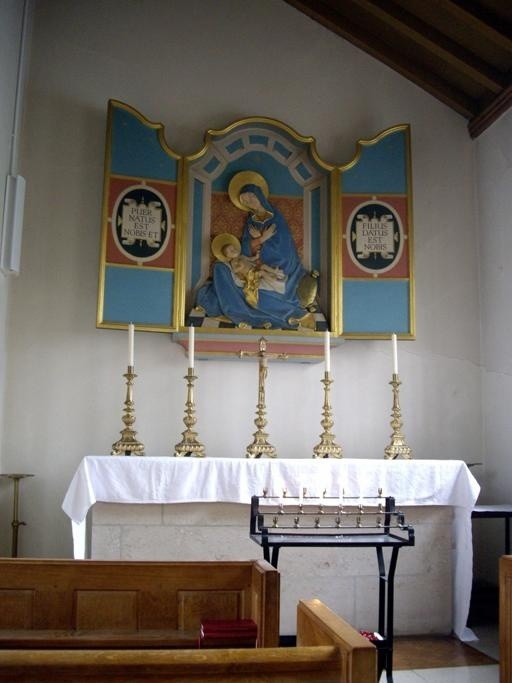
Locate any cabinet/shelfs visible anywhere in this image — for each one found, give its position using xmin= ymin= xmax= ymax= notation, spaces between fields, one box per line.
xmin=249 ymin=487 xmax=415 ymax=683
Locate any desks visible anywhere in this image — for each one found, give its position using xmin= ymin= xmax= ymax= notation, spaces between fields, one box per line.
xmin=61 ymin=456 xmax=481 ymax=643
xmin=472 ymin=505 xmax=512 ymax=556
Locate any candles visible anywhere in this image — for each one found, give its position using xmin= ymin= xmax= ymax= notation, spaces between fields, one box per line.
xmin=323 ymin=328 xmax=331 ymax=372
xmin=391 ymin=332 xmax=398 ymax=374
xmin=188 ymin=321 xmax=195 ymax=368
xmin=128 ymin=320 xmax=134 ymax=367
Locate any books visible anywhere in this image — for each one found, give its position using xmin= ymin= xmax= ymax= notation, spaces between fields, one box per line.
xmin=199 ymin=627 xmax=257 ymax=638
xmin=201 ymin=620 xmax=258 ymax=632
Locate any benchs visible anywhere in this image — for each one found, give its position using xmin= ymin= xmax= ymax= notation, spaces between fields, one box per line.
xmin=497 ymin=554 xmax=512 ymax=682
xmin=0 ymin=554 xmax=381 ymax=683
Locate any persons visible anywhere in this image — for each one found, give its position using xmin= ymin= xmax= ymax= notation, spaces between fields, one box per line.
xmin=196 ymin=182 xmax=313 ymax=328
xmin=223 ymin=240 xmax=285 ymax=287
